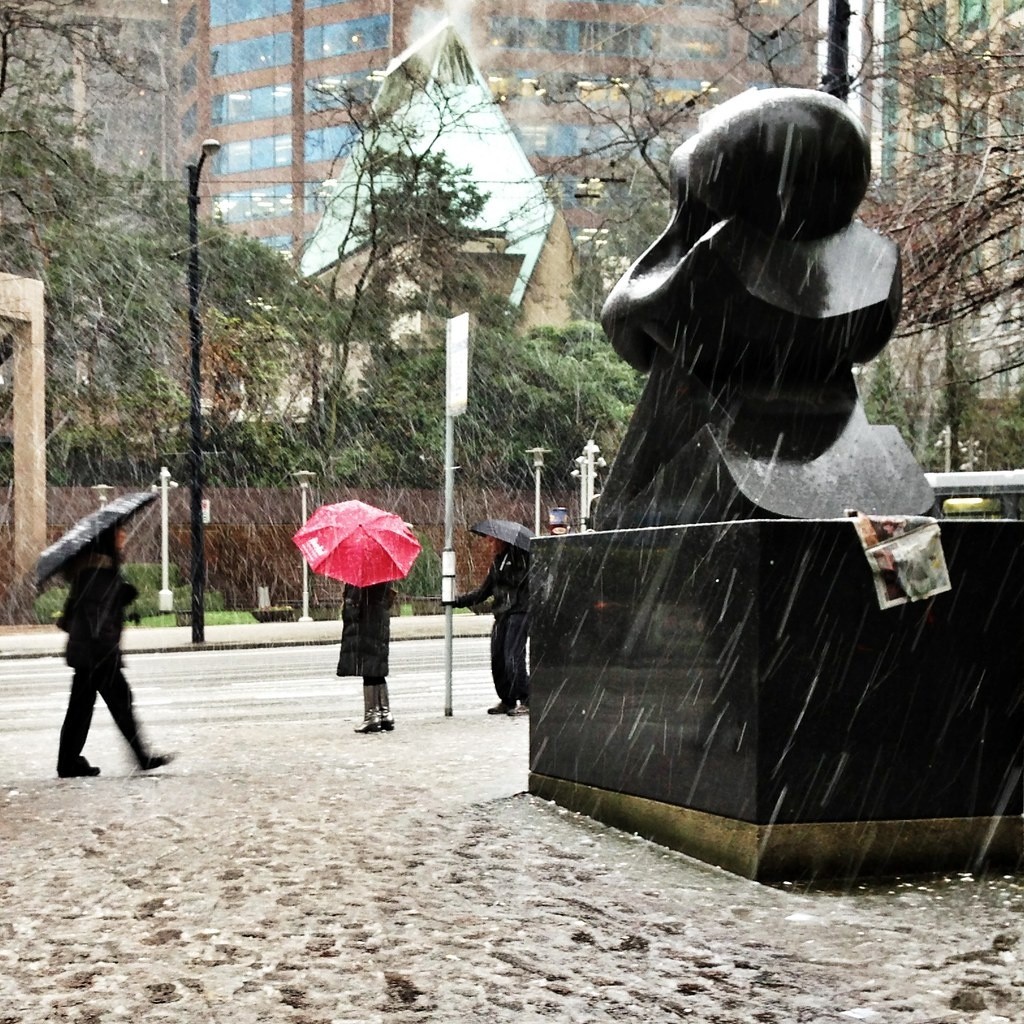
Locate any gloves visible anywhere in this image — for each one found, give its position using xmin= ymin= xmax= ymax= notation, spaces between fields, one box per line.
xmin=439 ymin=599 xmax=461 ymax=609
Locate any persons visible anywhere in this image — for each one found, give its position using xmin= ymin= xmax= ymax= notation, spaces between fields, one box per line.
xmin=291 ymin=499 xmax=422 ymax=589
xmin=336 ymin=578 xmax=397 ymax=733
xmin=439 ymin=533 xmax=532 ymax=717
xmin=56 ymin=520 xmax=168 ymax=778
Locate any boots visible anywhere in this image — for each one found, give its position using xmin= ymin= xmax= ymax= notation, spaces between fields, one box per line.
xmin=354 ymin=684 xmax=381 ymax=733
xmin=376 ymin=683 xmax=394 ymax=730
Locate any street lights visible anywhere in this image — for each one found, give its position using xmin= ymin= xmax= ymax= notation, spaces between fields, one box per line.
xmin=523 ymin=447 xmax=553 ymax=536
xmin=151 ymin=465 xmax=180 ymax=614
xmin=89 ymin=483 xmax=115 ymax=511
xmin=186 ymin=136 xmax=222 ymax=647
xmin=934 ymin=424 xmax=964 ymax=473
xmin=569 ymin=440 xmax=608 ymax=533
xmin=292 ymin=468 xmax=317 ymax=624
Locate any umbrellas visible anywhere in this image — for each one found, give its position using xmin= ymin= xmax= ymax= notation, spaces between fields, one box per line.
xmin=468 ymin=516 xmax=535 ymax=573
xmin=35 ymin=492 xmax=159 ymax=588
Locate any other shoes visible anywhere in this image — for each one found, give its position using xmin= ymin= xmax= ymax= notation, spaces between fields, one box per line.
xmin=507 ymin=703 xmax=530 ymax=716
xmin=142 ymin=753 xmax=172 ymax=771
xmin=57 ymin=765 xmax=100 ymax=778
xmin=487 ymin=700 xmax=517 ymax=714
